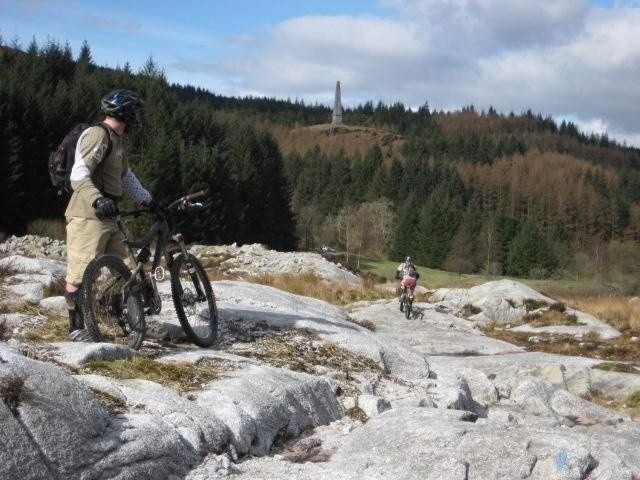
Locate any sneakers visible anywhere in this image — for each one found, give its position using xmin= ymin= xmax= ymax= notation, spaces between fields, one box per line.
xmin=67 ymin=326 xmax=98 ymax=342
xmin=129 ymin=327 xmax=169 ymax=342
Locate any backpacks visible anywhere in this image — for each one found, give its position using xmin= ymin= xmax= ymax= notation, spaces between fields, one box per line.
xmin=405 ymin=263 xmax=416 ymax=275
xmin=46 ymin=121 xmax=114 ymax=192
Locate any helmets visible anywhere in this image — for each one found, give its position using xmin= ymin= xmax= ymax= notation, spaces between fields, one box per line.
xmin=406 ymin=257 xmax=413 ymax=261
xmin=100 ymin=88 xmax=146 ymax=118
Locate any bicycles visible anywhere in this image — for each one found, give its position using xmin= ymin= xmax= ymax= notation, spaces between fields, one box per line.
xmin=395 ymin=275 xmax=415 ymax=319
xmin=76 ymin=181 xmax=221 ymax=353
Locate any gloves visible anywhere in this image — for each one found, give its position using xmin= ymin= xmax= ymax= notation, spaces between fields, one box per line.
xmin=92 ymin=195 xmax=119 ymax=222
xmin=149 ymin=199 xmax=171 ymax=218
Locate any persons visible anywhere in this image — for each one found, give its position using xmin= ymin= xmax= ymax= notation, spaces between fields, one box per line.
xmin=64 ymin=86 xmax=169 ymax=343
xmin=395 ymin=255 xmax=420 ymax=312
